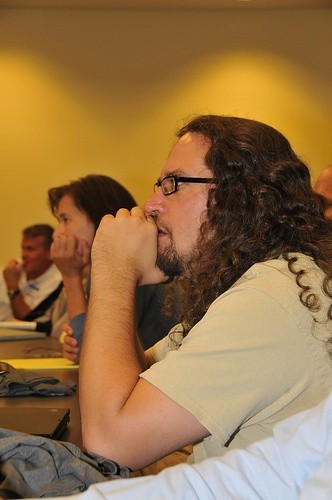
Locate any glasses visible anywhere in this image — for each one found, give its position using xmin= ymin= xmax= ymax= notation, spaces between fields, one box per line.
xmin=153 ymin=175 xmax=219 ymax=196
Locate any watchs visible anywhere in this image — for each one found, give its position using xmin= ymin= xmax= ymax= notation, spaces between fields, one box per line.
xmin=8 ymin=289 xmax=19 ymax=298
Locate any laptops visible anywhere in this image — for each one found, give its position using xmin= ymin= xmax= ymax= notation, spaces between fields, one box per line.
xmin=0 ymin=407 xmax=71 ymax=439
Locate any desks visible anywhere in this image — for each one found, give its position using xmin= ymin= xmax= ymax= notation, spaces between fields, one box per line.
xmin=0 ymin=336 xmax=82 ymax=452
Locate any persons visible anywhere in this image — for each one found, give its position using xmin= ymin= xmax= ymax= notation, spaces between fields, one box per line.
xmin=78 ymin=115 xmax=332 ymax=477
xmin=0 ymin=166 xmax=332 ymax=500
